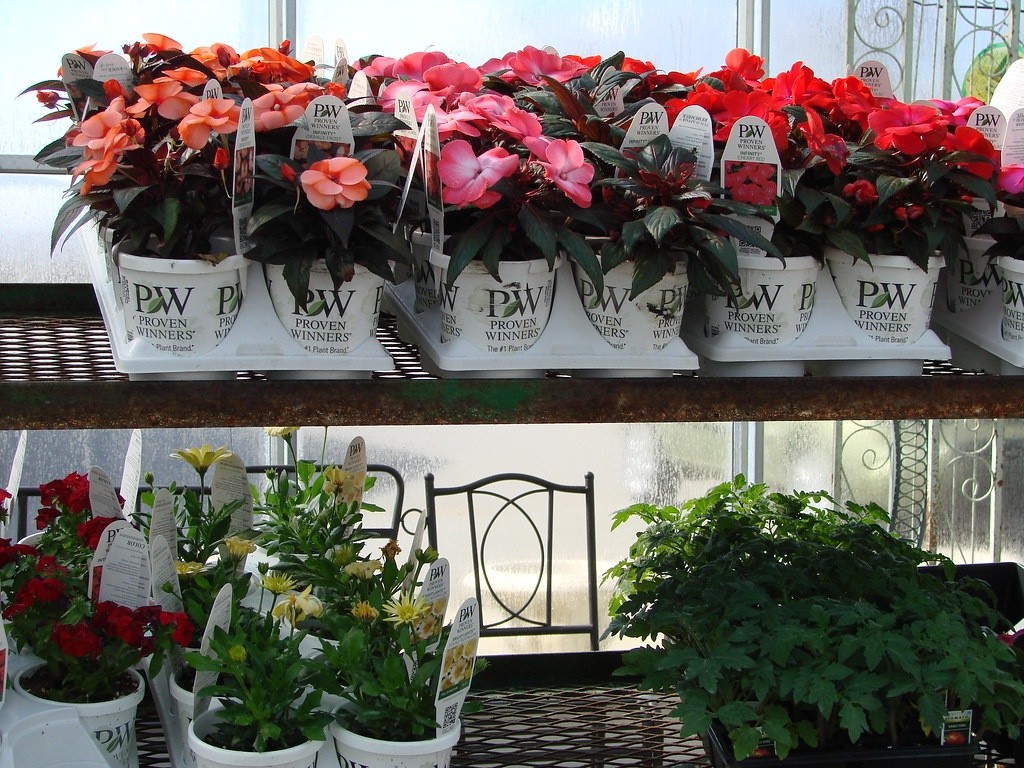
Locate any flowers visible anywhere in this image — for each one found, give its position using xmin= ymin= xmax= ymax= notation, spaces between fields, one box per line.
xmin=20 ymin=35 xmax=1024 ymax=312
xmin=0 ymin=425 xmax=490 ymax=747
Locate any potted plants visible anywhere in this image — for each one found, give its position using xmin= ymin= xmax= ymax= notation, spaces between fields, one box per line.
xmin=599 ymin=475 xmax=1023 ymax=768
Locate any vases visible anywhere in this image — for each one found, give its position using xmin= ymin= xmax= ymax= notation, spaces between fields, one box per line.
xmin=94 ymin=224 xmax=111 ymax=283
xmin=264 ymin=257 xmax=385 ymax=355
xmin=393 ymin=224 xmax=415 ymax=285
xmin=407 ymin=229 xmax=451 ymax=314
xmin=997 ymin=255 xmax=1024 ymax=342
xmin=328 ymin=697 xmax=460 ymax=768
xmin=112 ymin=234 xmax=252 ymax=358
xmin=567 ymin=252 xmax=688 ymax=354
xmin=948 ymin=236 xmax=1000 ymax=312
xmin=96 ymin=226 xmax=122 ymax=311
xmin=823 ymin=245 xmax=946 ymax=344
xmin=13 ymin=660 xmax=144 ymax=768
xmin=427 ymin=248 xmax=563 ymax=353
xmin=705 ymin=251 xmax=825 ymax=345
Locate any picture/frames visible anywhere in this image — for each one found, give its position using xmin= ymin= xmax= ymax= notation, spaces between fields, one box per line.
xmin=187 ymin=706 xmax=323 ymax=768
xmin=169 ymin=660 xmax=244 ymax=768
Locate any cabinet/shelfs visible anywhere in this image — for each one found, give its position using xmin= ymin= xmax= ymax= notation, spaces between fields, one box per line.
xmin=4 ymin=277 xmax=1022 ymax=768
